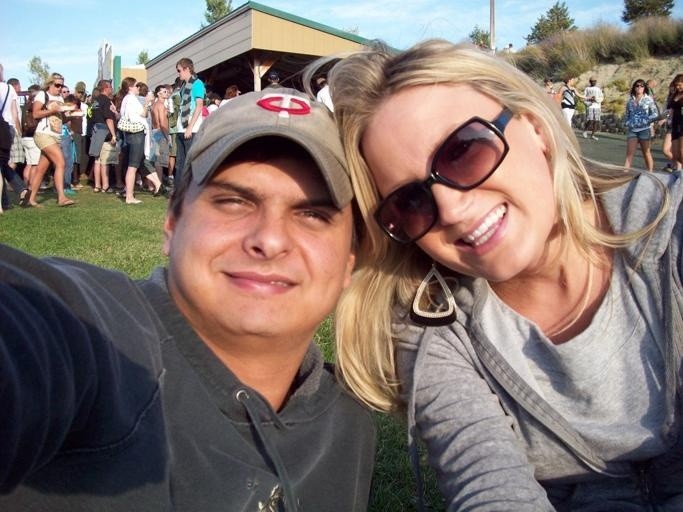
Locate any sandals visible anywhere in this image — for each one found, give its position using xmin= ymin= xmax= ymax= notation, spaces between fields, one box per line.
xmin=101 ymin=187 xmax=115 ymax=193
xmin=94 ymin=186 xmax=102 ymax=193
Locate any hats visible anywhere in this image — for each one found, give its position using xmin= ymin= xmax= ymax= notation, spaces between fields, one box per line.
xmin=183 ymin=88 xmax=354 ymax=209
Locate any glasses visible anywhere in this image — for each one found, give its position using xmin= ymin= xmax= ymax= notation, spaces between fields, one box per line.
xmin=54 ymin=83 xmax=65 ymax=89
xmin=78 ymin=91 xmax=84 ymax=94
xmin=176 ymin=69 xmax=182 ymax=73
xmin=63 ymin=90 xmax=71 ymax=94
xmin=372 ymin=107 xmax=520 ymax=244
xmin=634 ymin=84 xmax=644 ymax=87
xmin=131 ymin=84 xmax=140 ymax=88
xmin=163 ymin=90 xmax=169 ymax=93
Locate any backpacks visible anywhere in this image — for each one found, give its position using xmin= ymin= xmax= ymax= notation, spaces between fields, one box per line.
xmin=20 ymin=89 xmax=49 ymax=139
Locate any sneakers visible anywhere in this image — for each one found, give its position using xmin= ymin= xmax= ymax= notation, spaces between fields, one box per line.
xmin=167 ymin=175 xmax=174 ymax=184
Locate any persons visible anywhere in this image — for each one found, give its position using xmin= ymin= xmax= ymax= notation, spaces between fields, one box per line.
xmin=315 ymin=77 xmax=336 ymax=113
xmin=0 ymin=57 xmax=242 ymax=212
xmin=671 ymin=74 xmax=682 ymax=169
xmin=266 ymin=71 xmax=284 ymax=89
xmin=555 ymin=76 xmax=593 ymax=129
xmin=623 ymin=78 xmax=658 ymax=173
xmin=578 ymin=75 xmax=604 ymax=141
xmin=0 ymin=87 xmax=377 ymax=512
xmin=541 ymin=77 xmax=560 ymax=103
xmin=644 ymin=79 xmax=659 ymax=140
xmin=657 ymin=79 xmax=676 ymax=172
xmin=303 ymin=40 xmax=683 ymax=512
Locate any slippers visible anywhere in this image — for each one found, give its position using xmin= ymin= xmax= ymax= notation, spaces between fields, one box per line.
xmin=27 ymin=202 xmax=42 ymax=209
xmin=58 ymin=200 xmax=75 ymax=207
xmin=18 ymin=189 xmax=32 ymax=206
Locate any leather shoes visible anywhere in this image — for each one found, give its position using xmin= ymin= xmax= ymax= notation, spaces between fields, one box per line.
xmin=125 ymin=198 xmax=142 ymax=204
xmin=154 ymin=183 xmax=163 ymax=196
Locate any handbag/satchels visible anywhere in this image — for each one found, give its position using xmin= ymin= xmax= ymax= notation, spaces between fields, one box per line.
xmin=117 ymin=104 xmax=145 ymax=134
